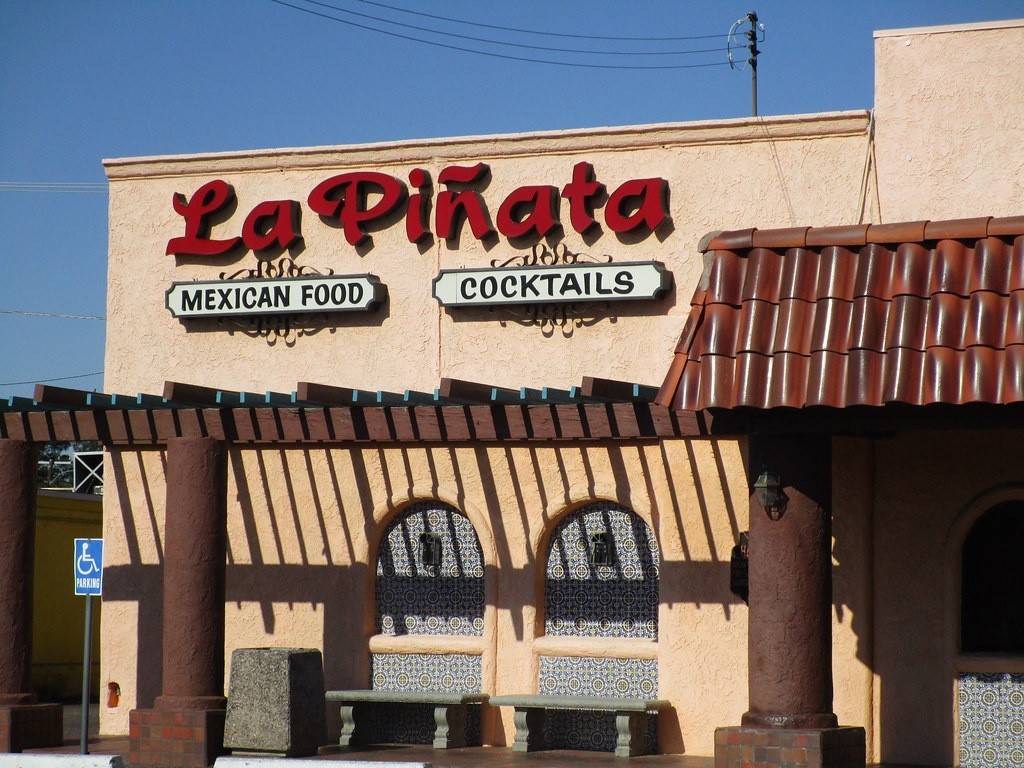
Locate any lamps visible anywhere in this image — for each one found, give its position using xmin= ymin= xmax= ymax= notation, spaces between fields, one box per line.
xmin=753 ymin=474 xmax=781 ymax=519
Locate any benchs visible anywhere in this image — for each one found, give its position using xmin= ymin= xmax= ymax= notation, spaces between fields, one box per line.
xmin=487 ymin=694 xmax=672 ymax=758
xmin=324 ymin=689 xmax=489 ymax=750
